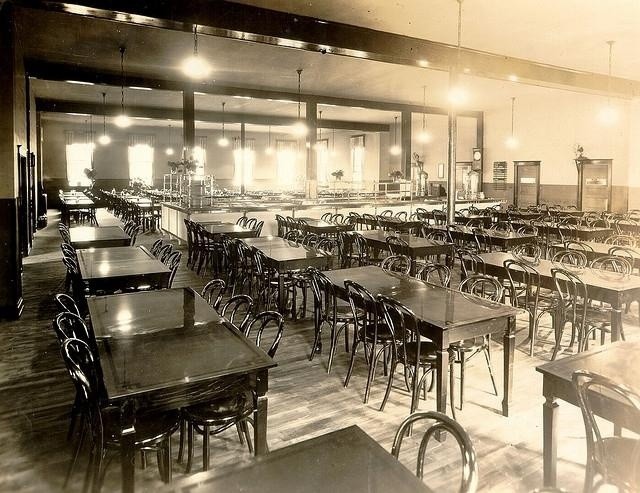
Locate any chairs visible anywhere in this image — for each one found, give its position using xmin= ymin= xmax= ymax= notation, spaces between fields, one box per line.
xmin=55 ymin=312 xmax=96 ymax=440
xmin=391 ymin=411 xmax=478 ymax=493
xmin=55 ymin=180 xmax=640 ymax=361
xmin=61 ymin=338 xmax=178 ymax=493
xmin=177 ymin=309 xmax=285 ymax=474
xmin=377 ymin=293 xmax=457 ymax=437
xmin=570 ymin=369 xmax=640 ymax=493
xmin=428 ymin=276 xmax=503 ymax=411
xmin=306 ymin=265 xmax=370 ymax=374
xmin=342 ymin=279 xmax=410 ymax=404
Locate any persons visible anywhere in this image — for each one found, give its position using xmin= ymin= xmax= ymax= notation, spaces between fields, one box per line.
xmin=437 ymin=183 xmax=446 ymax=197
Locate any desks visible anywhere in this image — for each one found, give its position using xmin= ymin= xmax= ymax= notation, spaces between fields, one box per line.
xmin=165 ymin=425 xmax=435 ymax=493
xmin=315 ymin=264 xmax=524 ymax=442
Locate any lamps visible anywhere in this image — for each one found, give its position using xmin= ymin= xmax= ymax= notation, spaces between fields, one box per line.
xmin=113 ymin=43 xmax=136 ymax=131
xmin=98 ymin=91 xmax=112 ymax=146
xmin=182 ymin=26 xmax=211 ymax=79
